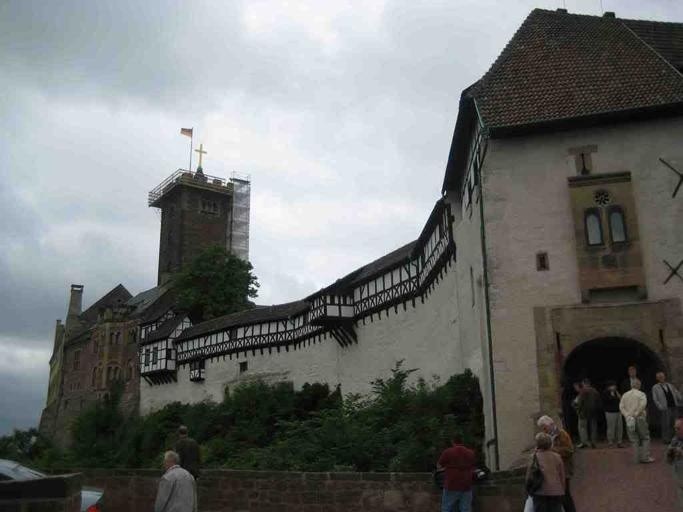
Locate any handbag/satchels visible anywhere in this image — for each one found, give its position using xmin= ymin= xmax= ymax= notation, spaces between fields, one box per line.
xmin=525 ymin=452 xmax=544 ymax=494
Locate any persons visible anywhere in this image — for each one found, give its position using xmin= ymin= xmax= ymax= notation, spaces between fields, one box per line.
xmin=436 ymin=433 xmax=477 ymax=512
xmin=572 ymin=365 xmax=682 ymax=510
xmin=524 ymin=432 xmax=566 ymax=512
xmin=537 ymin=415 xmax=577 ymax=512
xmin=153 ymin=450 xmax=195 ymax=511
xmin=168 ymin=425 xmax=202 ymax=510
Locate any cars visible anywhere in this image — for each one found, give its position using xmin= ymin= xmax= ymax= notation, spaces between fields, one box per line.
xmin=0 ymin=457 xmax=107 ymax=511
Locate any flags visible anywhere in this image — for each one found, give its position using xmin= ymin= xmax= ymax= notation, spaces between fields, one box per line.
xmin=181 ymin=128 xmax=192 ymax=137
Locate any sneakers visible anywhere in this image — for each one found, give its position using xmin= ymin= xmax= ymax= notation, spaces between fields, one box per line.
xmin=576 ymin=442 xmax=629 ymax=449
xmin=642 ymin=456 xmax=656 ymax=463
